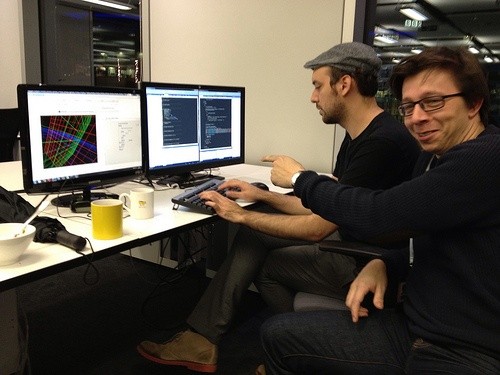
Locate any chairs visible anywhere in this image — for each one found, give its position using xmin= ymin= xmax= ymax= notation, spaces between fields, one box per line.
xmin=294 ymin=148 xmax=436 ymax=315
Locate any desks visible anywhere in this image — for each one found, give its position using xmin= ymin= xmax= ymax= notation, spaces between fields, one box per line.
xmin=0 ymin=161 xmax=331 ymax=292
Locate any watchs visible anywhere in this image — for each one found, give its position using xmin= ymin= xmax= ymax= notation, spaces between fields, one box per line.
xmin=291 ymin=171 xmax=304 ymax=187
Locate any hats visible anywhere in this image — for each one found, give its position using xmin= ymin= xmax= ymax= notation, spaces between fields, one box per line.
xmin=304 ymin=41 xmax=382 ymax=82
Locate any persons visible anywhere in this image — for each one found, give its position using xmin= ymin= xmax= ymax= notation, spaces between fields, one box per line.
xmin=136 ymin=41 xmax=422 ymax=372
xmin=261 ymin=45 xmax=500 ymax=375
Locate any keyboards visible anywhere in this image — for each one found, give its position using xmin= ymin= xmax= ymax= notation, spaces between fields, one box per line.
xmin=171 ymin=178 xmax=241 ymax=214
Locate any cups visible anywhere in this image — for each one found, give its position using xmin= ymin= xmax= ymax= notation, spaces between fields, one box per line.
xmin=90 ymin=199 xmax=123 ymax=241
xmin=119 ymin=188 xmax=154 ymax=220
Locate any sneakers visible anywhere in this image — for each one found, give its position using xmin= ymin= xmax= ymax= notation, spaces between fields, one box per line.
xmin=256 ymin=365 xmax=266 ymax=375
xmin=137 ymin=330 xmax=218 ymax=372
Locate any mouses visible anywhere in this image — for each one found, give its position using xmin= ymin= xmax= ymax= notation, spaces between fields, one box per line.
xmin=251 ymin=183 xmax=269 ymax=191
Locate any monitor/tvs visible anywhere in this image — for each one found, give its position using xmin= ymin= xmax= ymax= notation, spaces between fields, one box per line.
xmin=17 ymin=84 xmax=147 ymax=208
xmin=140 ymin=82 xmax=245 ymax=188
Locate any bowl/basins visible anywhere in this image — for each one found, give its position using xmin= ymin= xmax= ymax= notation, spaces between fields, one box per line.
xmin=0 ymin=223 xmax=37 ymax=266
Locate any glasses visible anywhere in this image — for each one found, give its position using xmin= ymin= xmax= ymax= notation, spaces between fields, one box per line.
xmin=397 ymin=92 xmax=466 ymax=117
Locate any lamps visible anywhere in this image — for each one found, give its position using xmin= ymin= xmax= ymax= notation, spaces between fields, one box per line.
xmin=82 ymin=0 xmax=137 ymax=11
xmin=396 ymin=4 xmax=433 ymax=24
